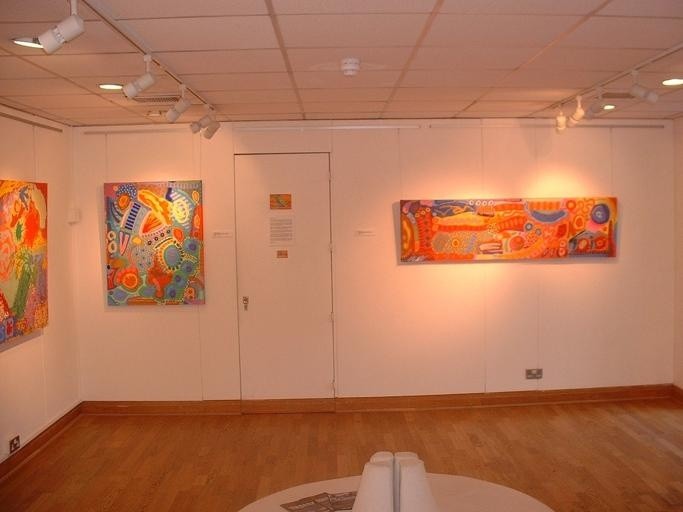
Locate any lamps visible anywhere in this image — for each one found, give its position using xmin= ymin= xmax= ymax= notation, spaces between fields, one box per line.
xmin=36 ymin=1 xmax=85 ymax=55
xmin=163 ymin=81 xmax=222 ymax=139
xmin=121 ymin=51 xmax=161 ymax=99
xmin=553 ymin=62 xmax=658 ymax=131
